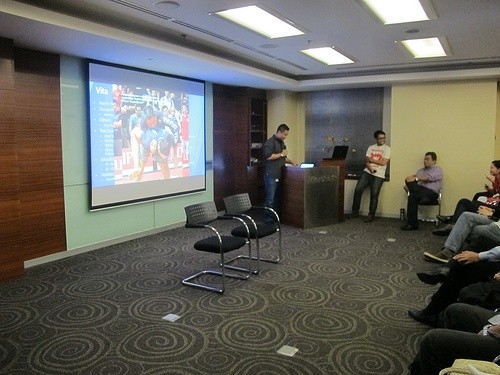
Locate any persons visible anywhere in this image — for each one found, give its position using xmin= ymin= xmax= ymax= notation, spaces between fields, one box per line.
xmin=262 ymin=124 xmax=299 ymax=223
xmin=401 ymin=152 xmax=443 ymax=232
xmin=408 ymin=159 xmax=500 ymax=375
xmin=113 ymin=84 xmax=189 ymax=184
xmin=347 ymin=130 xmax=392 ymax=223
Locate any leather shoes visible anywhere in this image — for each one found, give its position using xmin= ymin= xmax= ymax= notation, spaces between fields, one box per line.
xmin=408 ymin=309 xmax=440 ymax=328
xmin=417 ymin=267 xmax=450 ymax=284
xmin=437 ymin=216 xmax=454 ymax=224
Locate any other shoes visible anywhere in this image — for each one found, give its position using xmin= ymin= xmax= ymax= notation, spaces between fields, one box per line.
xmin=347 ymin=212 xmax=359 ymax=217
xmin=272 ymin=222 xmax=284 ymax=228
xmin=364 ymin=216 xmax=374 ymax=223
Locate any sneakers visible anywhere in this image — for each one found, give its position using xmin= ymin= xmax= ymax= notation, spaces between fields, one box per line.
xmin=423 ymin=249 xmax=454 ymax=263
xmin=400 ymin=225 xmax=419 ymax=230
xmin=432 ymin=224 xmax=452 ymax=236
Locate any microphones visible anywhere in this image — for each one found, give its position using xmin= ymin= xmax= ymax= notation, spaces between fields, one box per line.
xmin=371 ymin=170 xmax=377 ymax=173
xmin=283 ymin=145 xmax=286 ymax=150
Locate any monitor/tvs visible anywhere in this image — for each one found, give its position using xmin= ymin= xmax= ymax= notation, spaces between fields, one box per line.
xmin=300 ymin=162 xmax=315 ymax=168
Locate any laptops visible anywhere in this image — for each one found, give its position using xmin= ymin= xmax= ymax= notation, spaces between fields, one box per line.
xmin=323 ymin=145 xmax=349 ymax=161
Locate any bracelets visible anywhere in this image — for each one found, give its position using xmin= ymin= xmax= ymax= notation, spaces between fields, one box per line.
xmin=483 ymin=324 xmax=497 ymax=337
xmin=280 ymin=153 xmax=284 ymax=157
xmin=415 ymin=177 xmax=419 ymax=182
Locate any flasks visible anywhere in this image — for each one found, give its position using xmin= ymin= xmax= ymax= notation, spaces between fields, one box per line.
xmin=400 ymin=208 xmax=405 ymax=222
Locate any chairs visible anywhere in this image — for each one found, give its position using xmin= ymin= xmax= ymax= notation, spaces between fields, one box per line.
xmin=220 ymin=192 xmax=282 ymax=275
xmin=181 ymin=201 xmax=252 ymax=294
xmin=403 ymin=185 xmax=442 ymax=228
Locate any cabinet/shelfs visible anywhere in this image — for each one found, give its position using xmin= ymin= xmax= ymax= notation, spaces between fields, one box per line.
xmin=247 ymin=96 xmax=267 ymax=166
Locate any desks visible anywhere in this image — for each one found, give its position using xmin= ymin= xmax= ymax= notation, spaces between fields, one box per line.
xmin=280 ymin=166 xmax=340 ymax=229
